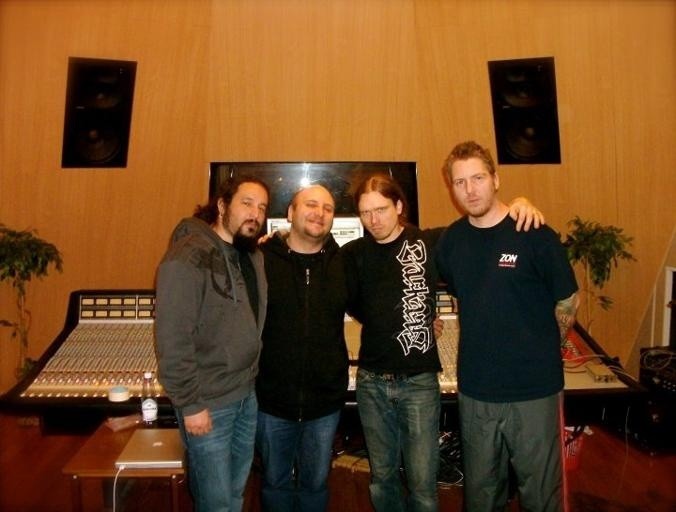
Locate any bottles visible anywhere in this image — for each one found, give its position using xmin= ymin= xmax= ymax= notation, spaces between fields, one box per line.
xmin=141 ymin=370 xmax=159 ymax=428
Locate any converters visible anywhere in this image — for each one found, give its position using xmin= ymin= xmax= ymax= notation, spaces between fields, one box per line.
xmin=586 ymin=365 xmax=617 ymax=383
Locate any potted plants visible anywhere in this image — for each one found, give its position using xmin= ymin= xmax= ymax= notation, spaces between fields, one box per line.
xmin=1 ymin=220 xmax=64 ymax=427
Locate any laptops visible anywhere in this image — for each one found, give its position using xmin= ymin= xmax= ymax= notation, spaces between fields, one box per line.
xmin=115 ymin=429 xmax=186 ymax=469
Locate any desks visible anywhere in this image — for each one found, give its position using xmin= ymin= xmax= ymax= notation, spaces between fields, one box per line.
xmin=62 ymin=416 xmax=186 ymax=512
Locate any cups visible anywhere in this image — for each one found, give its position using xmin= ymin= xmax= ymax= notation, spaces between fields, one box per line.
xmin=108 ymin=387 xmax=130 ymax=418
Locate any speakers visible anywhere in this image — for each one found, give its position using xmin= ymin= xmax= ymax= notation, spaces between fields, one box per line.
xmin=487 ymin=56 xmax=561 ymax=165
xmin=61 ymin=55 xmax=138 ymax=170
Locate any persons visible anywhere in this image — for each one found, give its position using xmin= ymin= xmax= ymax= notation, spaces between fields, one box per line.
xmin=156 ymin=174 xmax=270 ymax=512
xmin=433 ymin=140 xmax=580 ymax=512
xmin=256 ymin=175 xmax=545 ymax=512
xmin=257 ymin=185 xmax=443 ymax=512
